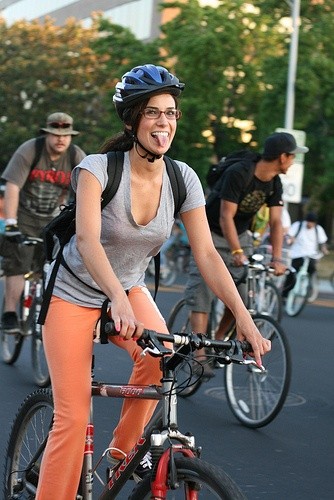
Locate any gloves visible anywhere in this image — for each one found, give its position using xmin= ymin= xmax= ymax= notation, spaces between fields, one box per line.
xmin=4 ymin=225 xmax=22 ymax=243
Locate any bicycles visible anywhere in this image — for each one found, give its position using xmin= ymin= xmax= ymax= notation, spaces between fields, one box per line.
xmin=0 ymin=230 xmax=325 ymax=429
xmin=1 ymin=322 xmax=268 ymax=500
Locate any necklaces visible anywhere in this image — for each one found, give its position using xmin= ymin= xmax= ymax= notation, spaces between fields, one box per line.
xmin=46 ymin=151 xmax=62 ymax=172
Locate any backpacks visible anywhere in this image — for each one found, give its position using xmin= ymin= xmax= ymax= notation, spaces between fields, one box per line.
xmin=206 ymin=151 xmax=261 ymax=190
xmin=43 ymin=150 xmax=187 ymax=263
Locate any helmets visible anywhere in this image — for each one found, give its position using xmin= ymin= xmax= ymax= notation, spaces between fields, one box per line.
xmin=113 ymin=64 xmax=185 ymax=102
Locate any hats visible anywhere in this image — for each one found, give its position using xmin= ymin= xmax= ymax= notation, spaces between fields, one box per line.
xmin=41 ymin=113 xmax=79 ymax=135
xmin=306 ymin=213 xmax=318 ymax=221
xmin=265 ymin=133 xmax=309 ymax=155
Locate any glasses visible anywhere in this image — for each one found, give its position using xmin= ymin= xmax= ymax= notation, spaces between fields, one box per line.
xmin=141 ymin=107 xmax=181 ymax=119
xmin=48 ymin=122 xmax=72 ymax=128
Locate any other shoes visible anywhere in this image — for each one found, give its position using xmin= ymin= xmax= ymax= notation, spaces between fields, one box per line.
xmin=281 ymin=297 xmax=287 ymax=305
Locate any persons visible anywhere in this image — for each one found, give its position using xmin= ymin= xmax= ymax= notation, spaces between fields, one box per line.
xmin=185 ymin=133 xmax=310 ymax=377
xmin=282 ymin=212 xmax=331 ymax=306
xmin=160 ymin=217 xmax=195 ymax=279
xmin=33 ymin=65 xmax=272 ymax=500
xmin=2 ymin=112 xmax=88 ymax=335
xmin=250 ymin=199 xmax=295 ymax=267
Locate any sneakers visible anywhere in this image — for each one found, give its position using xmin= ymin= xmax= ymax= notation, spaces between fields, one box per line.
xmin=107 ymin=445 xmax=152 ymax=484
xmin=213 ymin=347 xmax=226 ymax=368
xmin=0 ymin=311 xmax=20 ymax=332
xmin=192 ymin=355 xmax=216 ymax=377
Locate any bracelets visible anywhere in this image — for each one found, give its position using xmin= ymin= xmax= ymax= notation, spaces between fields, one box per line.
xmin=271 ymin=258 xmax=282 ymax=262
xmin=4 ymin=218 xmax=17 ymax=226
xmin=232 ymin=249 xmax=244 ymax=255
